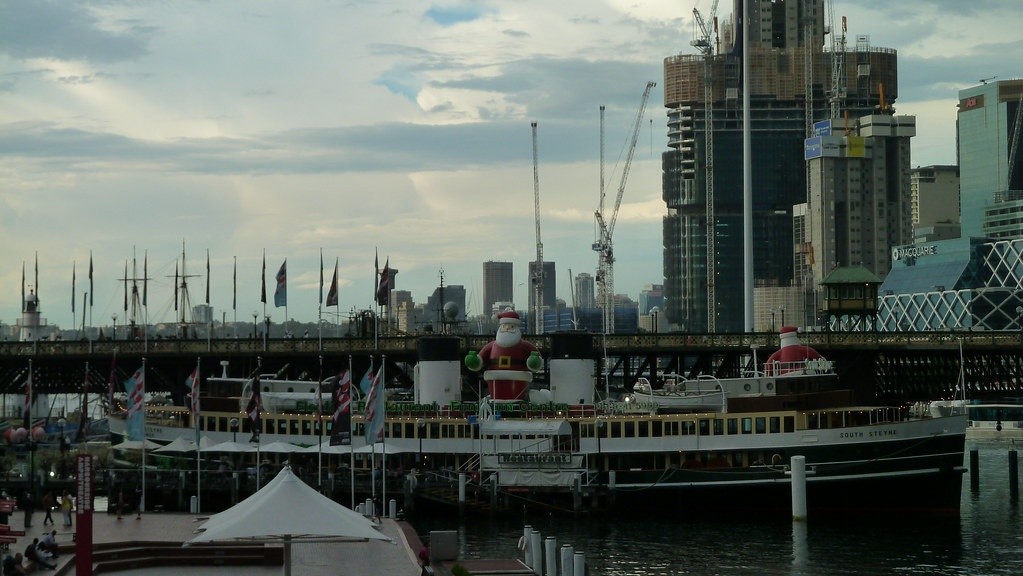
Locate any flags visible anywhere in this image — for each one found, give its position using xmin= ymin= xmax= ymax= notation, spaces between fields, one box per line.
xmin=246 ymin=372 xmax=259 ymax=431
xmin=124 ymin=367 xmax=144 ymax=420
xmin=275 ymin=253 xmax=388 ymax=312
xmin=331 ymin=370 xmax=351 ymax=426
xmin=360 ymin=365 xmax=373 ymax=397
xmin=364 ymin=365 xmax=383 ymax=446
xmin=185 ymin=367 xmax=200 ymax=417
xmin=23 ymin=375 xmax=31 ymax=429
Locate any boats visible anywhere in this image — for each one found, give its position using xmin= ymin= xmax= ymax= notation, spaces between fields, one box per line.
xmin=106 ymin=338 xmax=968 ymax=507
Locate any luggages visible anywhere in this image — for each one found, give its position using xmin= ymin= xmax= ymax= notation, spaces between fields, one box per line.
xmin=423 ymin=565 xmax=434 ymax=576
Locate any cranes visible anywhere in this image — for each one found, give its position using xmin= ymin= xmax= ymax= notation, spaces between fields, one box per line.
xmin=591 ymin=81 xmax=657 ymax=333
xmin=531 ymin=121 xmax=545 ymax=335
xmin=825 ymin=0 xmax=847 ymax=119
xmin=688 ymin=0 xmax=718 ymax=333
xmin=805 ymin=0 xmax=814 ymax=212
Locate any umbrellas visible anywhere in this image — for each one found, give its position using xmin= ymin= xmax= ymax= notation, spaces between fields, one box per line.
xmin=177 ymin=464 xmax=399 ymax=576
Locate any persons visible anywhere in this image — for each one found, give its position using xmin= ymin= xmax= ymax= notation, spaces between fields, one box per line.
xmin=44 ymin=490 xmax=54 ymax=525
xmin=3 ymin=553 xmax=30 ymax=576
xmin=40 ymin=530 xmax=59 ymax=559
xmin=25 ymin=538 xmax=57 ymax=570
xmin=23 ymin=491 xmax=34 ymax=528
xmin=61 ymin=490 xmax=73 ymax=526
xmin=465 ymin=307 xmax=545 ymax=399
